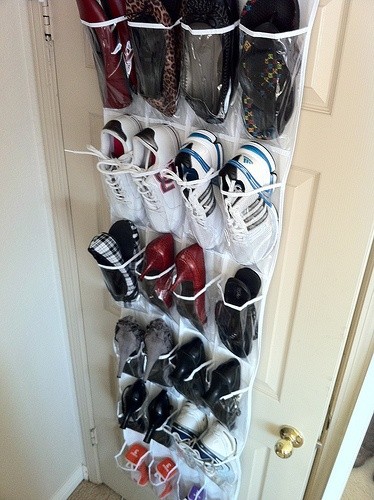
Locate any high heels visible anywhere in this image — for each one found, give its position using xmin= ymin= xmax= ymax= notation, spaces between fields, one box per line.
xmin=63 ymin=0 xmax=305 ymax=500
